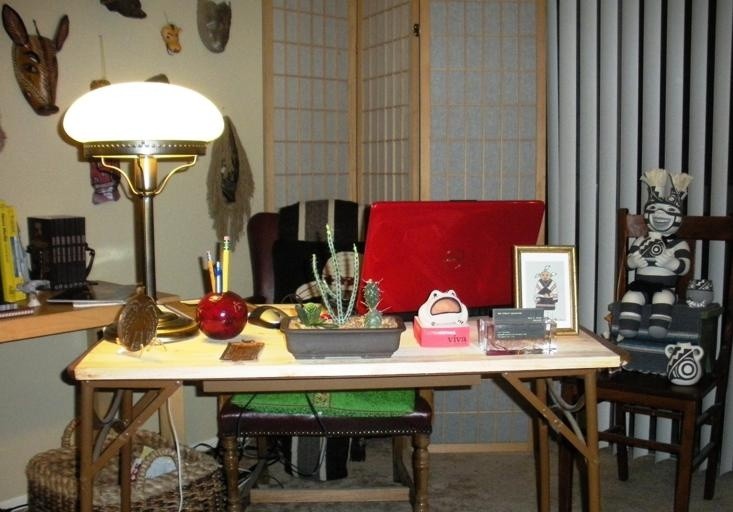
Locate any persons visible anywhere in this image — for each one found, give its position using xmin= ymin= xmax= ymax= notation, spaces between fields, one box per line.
xmin=616 ymin=164 xmax=692 ymax=341
xmin=532 ymin=264 xmax=560 ymax=323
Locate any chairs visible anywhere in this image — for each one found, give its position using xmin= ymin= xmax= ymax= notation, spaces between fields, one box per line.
xmin=560 ymin=208 xmax=733 ymax=512
xmin=219 ymin=199 xmax=433 ymax=512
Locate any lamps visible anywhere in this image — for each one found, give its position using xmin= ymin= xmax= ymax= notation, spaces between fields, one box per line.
xmin=62 ymin=81 xmax=223 ymax=345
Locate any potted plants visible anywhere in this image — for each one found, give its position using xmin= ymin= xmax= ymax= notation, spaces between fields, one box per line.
xmin=281 ymin=225 xmax=406 ymax=358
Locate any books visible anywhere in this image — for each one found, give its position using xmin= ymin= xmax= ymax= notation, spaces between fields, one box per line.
xmin=0 ymin=200 xmax=137 ymax=320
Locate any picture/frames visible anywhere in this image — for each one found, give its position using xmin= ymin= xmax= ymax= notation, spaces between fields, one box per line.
xmin=515 ymin=245 xmax=578 ymax=335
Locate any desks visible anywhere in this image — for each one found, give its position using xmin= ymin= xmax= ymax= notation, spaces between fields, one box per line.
xmin=0 ymin=279 xmax=186 ymax=451
xmin=67 ymin=303 xmax=632 ymax=512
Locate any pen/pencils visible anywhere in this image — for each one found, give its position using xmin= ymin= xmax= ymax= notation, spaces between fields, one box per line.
xmin=198 ymin=235 xmax=231 ymax=293
